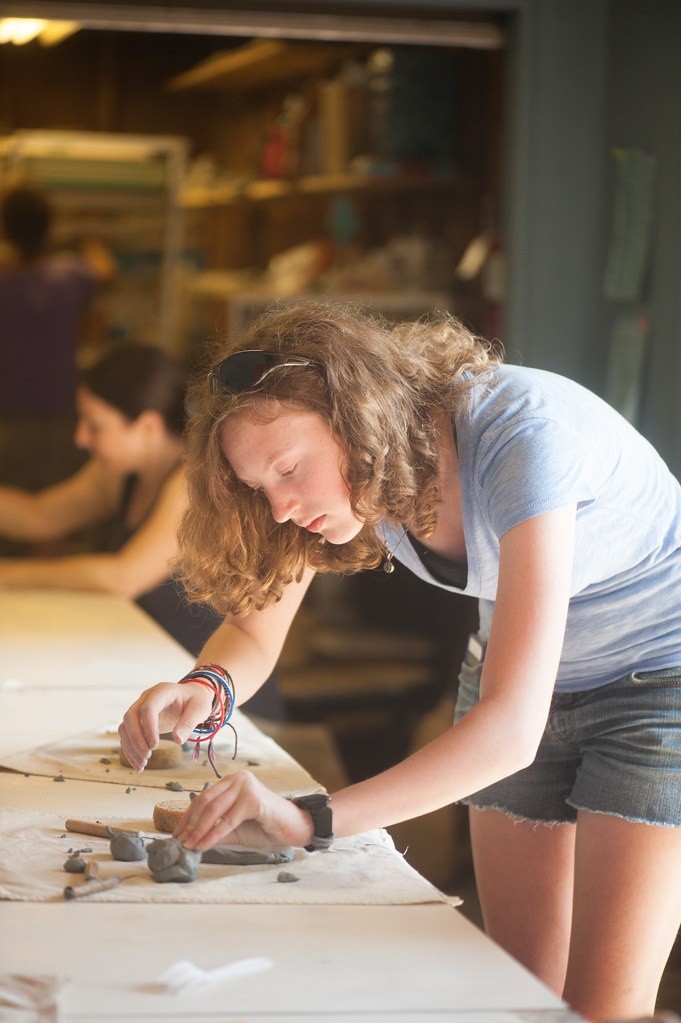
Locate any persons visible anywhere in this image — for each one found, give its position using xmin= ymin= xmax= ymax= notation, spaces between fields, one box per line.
xmin=116 ymin=303 xmax=681 ymax=1023
xmin=2 ymin=189 xmax=105 ymax=557
xmin=0 ymin=342 xmax=225 ymax=658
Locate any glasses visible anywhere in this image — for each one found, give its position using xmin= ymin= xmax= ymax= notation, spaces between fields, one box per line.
xmin=206 ymin=350 xmax=325 ymax=398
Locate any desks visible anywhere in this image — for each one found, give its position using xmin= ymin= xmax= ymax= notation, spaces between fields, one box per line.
xmin=0 ymin=588 xmax=591 ymax=1022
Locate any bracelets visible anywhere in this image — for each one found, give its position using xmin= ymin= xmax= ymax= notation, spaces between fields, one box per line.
xmin=291 ymin=793 xmax=334 ymax=853
xmin=177 ymin=662 xmax=238 ymax=779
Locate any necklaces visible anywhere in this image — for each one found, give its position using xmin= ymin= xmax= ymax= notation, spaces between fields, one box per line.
xmin=375 ymin=511 xmax=416 ymax=574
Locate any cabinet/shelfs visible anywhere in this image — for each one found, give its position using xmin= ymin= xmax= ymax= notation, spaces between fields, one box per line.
xmin=164 ymin=39 xmax=501 ymax=697
